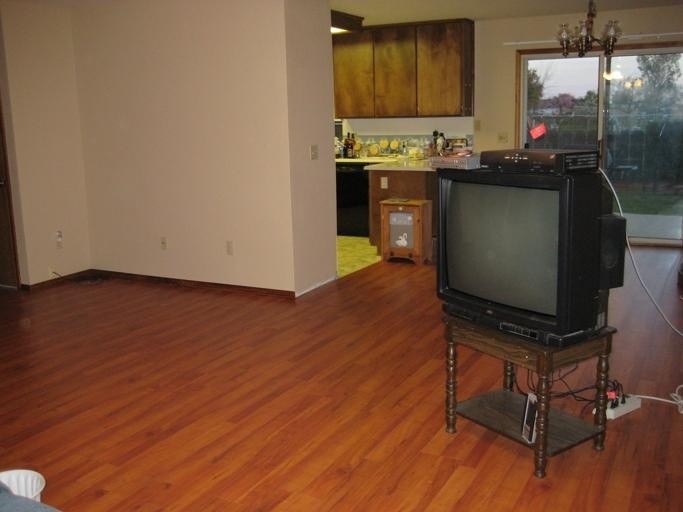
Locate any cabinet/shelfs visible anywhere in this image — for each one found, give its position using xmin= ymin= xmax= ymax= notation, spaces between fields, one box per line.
xmin=336 ymin=157 xmax=475 ymax=264
xmin=333 ymin=19 xmax=477 ymax=119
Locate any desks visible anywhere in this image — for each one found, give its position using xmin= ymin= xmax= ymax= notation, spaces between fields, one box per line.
xmin=442 ymin=316 xmax=617 ymax=478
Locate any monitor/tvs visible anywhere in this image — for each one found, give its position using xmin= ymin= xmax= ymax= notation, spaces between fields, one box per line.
xmin=436 ymin=170 xmax=626 ymax=337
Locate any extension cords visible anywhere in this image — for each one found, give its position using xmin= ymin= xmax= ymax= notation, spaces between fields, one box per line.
xmin=591 ymin=394 xmax=641 ymax=420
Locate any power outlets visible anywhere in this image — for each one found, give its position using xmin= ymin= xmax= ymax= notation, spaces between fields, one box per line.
xmin=160 ymin=237 xmax=169 ymax=253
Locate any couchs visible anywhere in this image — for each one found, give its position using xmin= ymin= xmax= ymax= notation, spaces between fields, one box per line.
xmin=0 ymin=481 xmax=60 ymax=512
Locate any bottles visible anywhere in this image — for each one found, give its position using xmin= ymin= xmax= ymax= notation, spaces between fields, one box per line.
xmin=343 ymin=132 xmax=356 ymax=158
xmin=430 ymin=130 xmax=446 ymax=156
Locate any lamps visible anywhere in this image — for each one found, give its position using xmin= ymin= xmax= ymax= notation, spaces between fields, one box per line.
xmin=557 ymin=1 xmax=619 ymax=56
